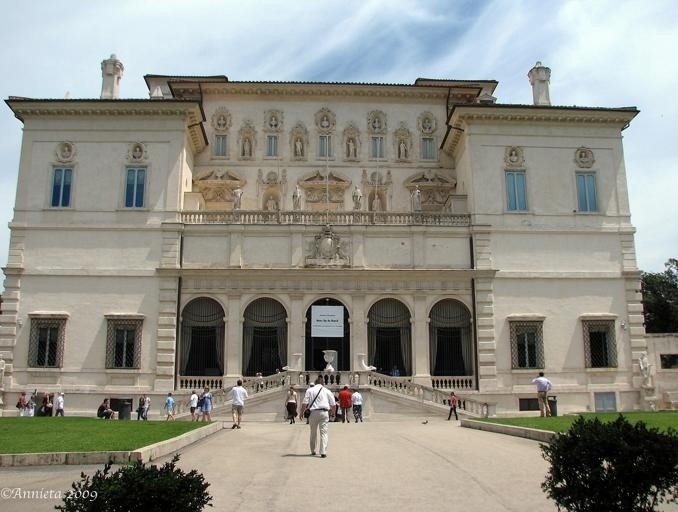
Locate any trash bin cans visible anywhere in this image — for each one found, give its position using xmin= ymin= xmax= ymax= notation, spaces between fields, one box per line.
xmin=547 ymin=396 xmax=558 ymax=418
xmin=119 ymin=402 xmax=131 ymax=419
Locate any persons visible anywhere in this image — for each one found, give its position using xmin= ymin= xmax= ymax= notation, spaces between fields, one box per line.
xmin=266 ymin=195 xmax=276 ymax=210
xmin=232 ymin=186 xmax=243 ymax=209
xmin=19 ymin=392 xmax=65 ymax=417
xmin=295 ymin=138 xmax=302 ymax=156
xmin=372 ymin=194 xmax=384 ymax=222
xmin=530 ymin=372 xmax=552 ymax=417
xmin=351 ymin=185 xmax=362 ymax=211
xmin=97 ymin=386 xmax=212 ymax=422
xmin=411 ymin=185 xmax=422 ymax=212
xmin=256 ymin=368 xmax=363 ymax=458
xmin=244 ymin=139 xmax=251 ymax=156
xmin=347 ymin=140 xmax=355 ymax=157
xmin=446 ymin=392 xmax=458 ymax=420
xmin=390 ymin=365 xmax=400 ymax=376
xmin=227 ymin=379 xmax=248 ymax=429
xmin=292 ymin=185 xmax=302 ymax=210
xmin=400 ymin=141 xmax=407 ymax=158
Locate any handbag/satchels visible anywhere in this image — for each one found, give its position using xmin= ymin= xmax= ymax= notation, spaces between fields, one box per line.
xmin=304 ymin=409 xmax=310 ymax=419
